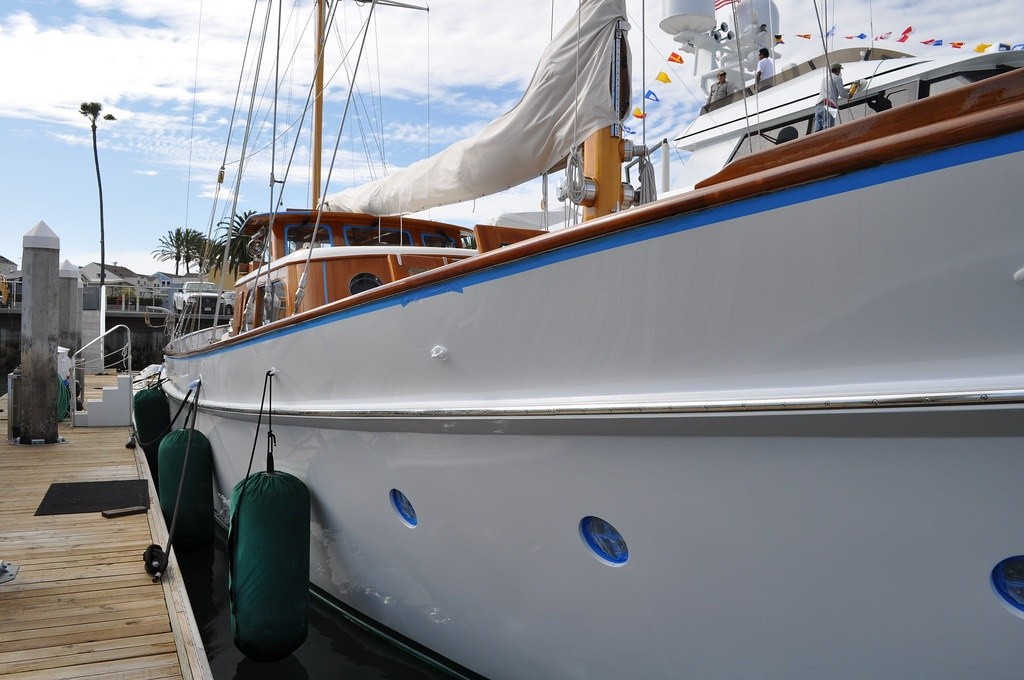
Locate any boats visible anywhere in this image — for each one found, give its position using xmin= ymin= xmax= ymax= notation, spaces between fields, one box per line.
xmin=155 ymin=1 xmax=1022 ymax=678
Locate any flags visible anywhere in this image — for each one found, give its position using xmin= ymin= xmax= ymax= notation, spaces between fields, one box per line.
xmin=774 ymin=23 xmax=992 ymax=53
xmin=622 ymin=50 xmax=685 ymax=137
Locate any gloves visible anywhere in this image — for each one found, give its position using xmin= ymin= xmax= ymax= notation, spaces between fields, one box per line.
xmin=849 ymin=83 xmax=858 ymax=97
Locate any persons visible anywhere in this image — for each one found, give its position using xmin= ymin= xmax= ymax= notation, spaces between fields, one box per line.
xmin=815 ymin=62 xmax=853 ymax=132
xmin=754 ymin=47 xmax=773 ymax=93
xmin=705 ymin=69 xmax=734 ymax=111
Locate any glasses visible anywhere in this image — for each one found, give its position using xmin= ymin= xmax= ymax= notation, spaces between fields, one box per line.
xmin=720 ymin=74 xmax=725 ymax=76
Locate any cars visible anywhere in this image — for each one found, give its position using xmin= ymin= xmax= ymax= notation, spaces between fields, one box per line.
xmin=171 ymin=282 xmax=241 ymax=315
xmin=0 ymin=273 xmax=11 ymax=309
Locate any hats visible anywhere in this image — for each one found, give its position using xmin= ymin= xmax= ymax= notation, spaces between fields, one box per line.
xmin=716 ymin=70 xmax=726 ymax=75
xmin=830 ymin=62 xmax=844 ymax=69
